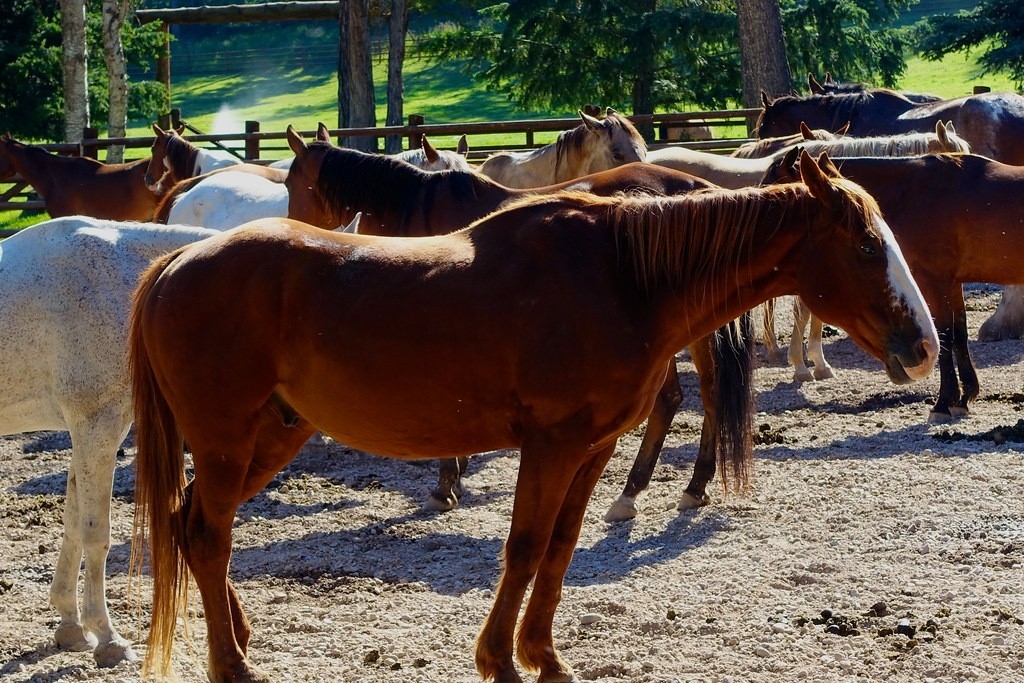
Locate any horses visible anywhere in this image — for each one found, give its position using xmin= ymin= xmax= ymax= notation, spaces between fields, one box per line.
xmin=1 ymin=73 xmax=1024 ymax=681
